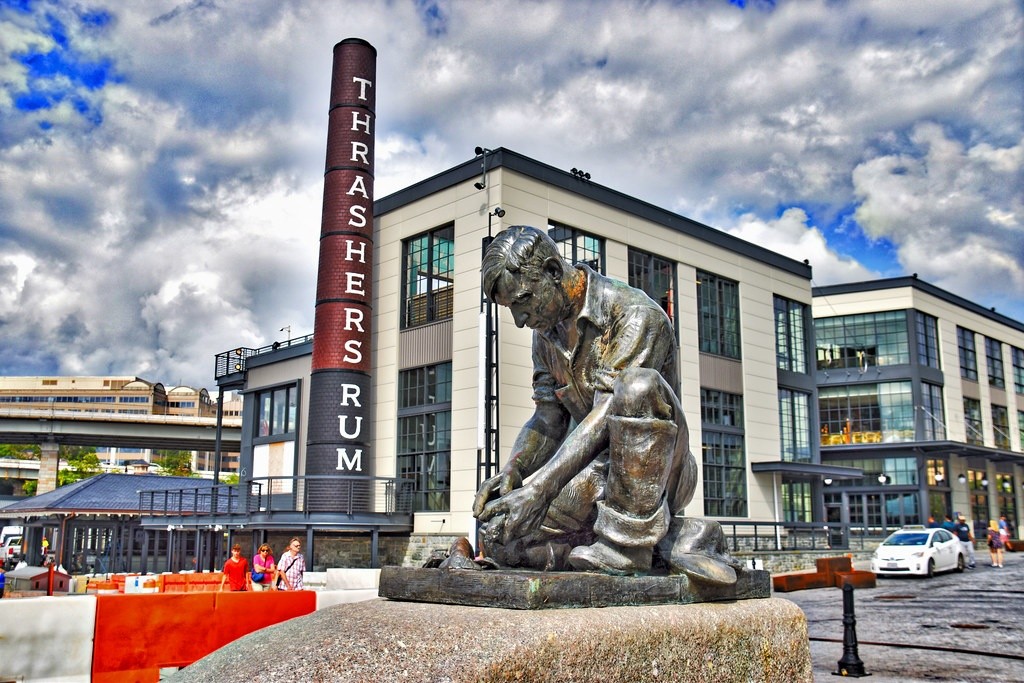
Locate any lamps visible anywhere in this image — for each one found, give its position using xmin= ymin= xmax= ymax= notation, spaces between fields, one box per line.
xmin=235 ymin=349 xmax=243 ymax=372
xmin=878 ymin=473 xmax=887 ymax=484
xmin=804 ymin=259 xmax=808 ymax=265
xmin=935 ymin=471 xmax=943 ymax=482
xmin=272 ymin=342 xmax=280 ymax=350
xmin=570 ymin=168 xmax=591 ymax=180
xmin=1002 ymin=478 xmax=1010 ymax=488
xmin=491 ymin=207 xmax=505 ymax=219
xmin=823 ymin=474 xmax=833 ymax=485
xmin=473 ymin=146 xmax=493 ymax=191
xmin=981 ymin=476 xmax=989 ymax=486
xmin=958 ymin=473 xmax=966 ymax=484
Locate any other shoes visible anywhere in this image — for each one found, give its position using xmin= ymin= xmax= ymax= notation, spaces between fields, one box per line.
xmin=990 ymin=564 xmax=998 ymax=567
xmin=969 ymin=564 xmax=976 ymax=568
xmin=999 ymin=564 xmax=1003 ymax=568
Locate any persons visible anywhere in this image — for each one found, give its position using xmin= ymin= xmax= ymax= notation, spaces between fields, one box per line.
xmin=472 ymin=225 xmax=742 ymax=575
xmin=8 ymin=552 xmax=27 ymax=571
xmin=987 ymin=519 xmax=1003 ymax=568
xmin=0 ymin=568 xmax=6 ymax=599
xmin=220 ymin=544 xmax=249 ymax=592
xmin=953 ymin=516 xmax=976 ymax=568
xmin=929 ymin=515 xmax=939 ymax=528
xmin=251 ymin=543 xmax=275 ymax=592
xmin=999 ymin=516 xmax=1015 ymax=552
xmin=940 ymin=514 xmax=956 ymax=533
xmin=276 ymin=538 xmax=305 ymax=591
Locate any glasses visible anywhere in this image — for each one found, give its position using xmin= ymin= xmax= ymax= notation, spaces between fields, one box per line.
xmin=294 ymin=545 xmax=302 ymax=547
xmin=261 ymin=550 xmax=269 ymax=552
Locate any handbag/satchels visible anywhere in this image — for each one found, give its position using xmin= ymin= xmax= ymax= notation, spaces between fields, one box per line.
xmin=251 ymin=569 xmax=264 ymax=582
xmin=276 ymin=575 xmax=282 ymax=586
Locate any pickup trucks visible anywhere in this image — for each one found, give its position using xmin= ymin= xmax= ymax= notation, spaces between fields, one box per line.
xmin=0 ymin=536 xmax=24 ymax=571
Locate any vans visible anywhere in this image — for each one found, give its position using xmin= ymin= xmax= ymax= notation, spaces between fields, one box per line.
xmin=0 ymin=525 xmax=24 ymax=547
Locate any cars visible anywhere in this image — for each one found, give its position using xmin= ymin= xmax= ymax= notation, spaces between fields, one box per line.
xmin=869 ymin=528 xmax=967 ymax=579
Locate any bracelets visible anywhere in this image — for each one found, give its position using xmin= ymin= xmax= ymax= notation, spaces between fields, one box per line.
xmin=265 ymin=569 xmax=266 ymax=571
